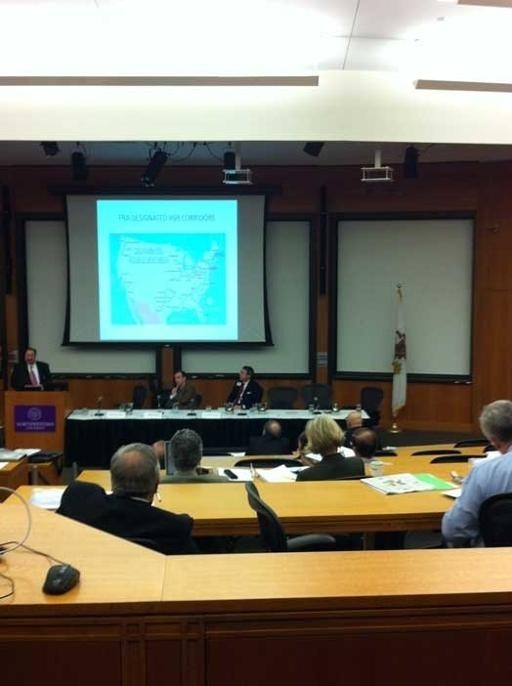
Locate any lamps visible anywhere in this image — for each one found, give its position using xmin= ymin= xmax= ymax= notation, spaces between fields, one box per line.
xmin=42 ymin=141 xmax=58 ymax=155
xmin=140 ymin=151 xmax=169 ymax=189
xmin=303 ymin=141 xmax=325 ymax=157
xmin=404 ymin=146 xmax=420 ymax=179
xmin=70 ymin=151 xmax=86 ymax=182
xmin=224 ymin=151 xmax=235 ymax=171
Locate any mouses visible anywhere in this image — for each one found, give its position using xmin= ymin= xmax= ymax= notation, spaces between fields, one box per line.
xmin=43 ymin=565 xmax=79 ymax=594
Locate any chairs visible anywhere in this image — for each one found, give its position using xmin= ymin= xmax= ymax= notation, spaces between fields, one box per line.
xmin=342 ymin=386 xmax=385 ymax=426
xmin=429 ymin=455 xmax=487 ymax=464
xmin=375 ymin=452 xmax=398 ymax=456
xmin=328 ymin=475 xmax=372 ymax=481
xmin=302 ymin=383 xmax=332 ymax=409
xmin=123 ymin=536 xmax=163 ymax=557
xmin=477 ymin=492 xmax=512 ymax=549
xmin=454 ymin=438 xmax=490 ymax=448
xmin=483 ymin=445 xmax=496 ymax=453
xmin=412 ymin=450 xmax=462 ymax=455
xmin=133 ymin=383 xmax=147 ymax=411
xmin=152 ymin=388 xmax=169 ymax=409
xmin=267 ymin=386 xmax=298 ymax=409
xmin=245 ymin=480 xmax=338 ymax=554
xmin=194 ymin=394 xmax=202 ymax=409
xmin=234 ymin=458 xmax=303 ymax=469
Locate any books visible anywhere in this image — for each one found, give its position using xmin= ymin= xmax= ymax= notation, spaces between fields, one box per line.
xmin=0 ymin=452 xmax=28 ymax=462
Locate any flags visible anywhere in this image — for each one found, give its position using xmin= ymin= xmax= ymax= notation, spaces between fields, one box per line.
xmin=391 ymin=291 xmax=408 ymax=418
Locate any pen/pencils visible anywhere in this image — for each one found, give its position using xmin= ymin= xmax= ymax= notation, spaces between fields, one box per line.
xmin=156 ymin=490 xmax=165 ymax=503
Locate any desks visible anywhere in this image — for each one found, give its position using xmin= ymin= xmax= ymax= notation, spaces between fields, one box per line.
xmin=388 ymin=443 xmax=453 ymax=452
xmin=71 ymin=462 xmax=477 ymax=480
xmin=200 ymin=453 xmax=442 ymax=467
xmin=27 ymin=459 xmax=60 ymax=486
xmin=0 ymin=479 xmax=468 ymax=541
xmin=63 ymin=408 xmax=372 ymax=468
xmin=0 ymin=455 xmax=32 ymax=502
xmin=0 ymin=503 xmax=512 ymax=686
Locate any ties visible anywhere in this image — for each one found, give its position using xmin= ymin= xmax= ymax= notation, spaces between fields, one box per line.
xmin=30 ymin=366 xmax=38 ymax=384
xmin=237 ymin=384 xmax=244 ymax=402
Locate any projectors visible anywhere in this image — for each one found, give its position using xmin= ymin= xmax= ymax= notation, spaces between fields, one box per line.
xmin=223 ymin=143 xmax=251 ymax=184
xmin=362 ymin=141 xmax=393 ymax=180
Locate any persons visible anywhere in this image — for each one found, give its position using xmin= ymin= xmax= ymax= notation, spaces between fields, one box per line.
xmin=295 ymin=414 xmax=365 ymax=481
xmin=164 ymin=370 xmax=194 ymax=410
xmin=10 ymin=347 xmax=54 ymax=392
xmin=440 ymin=399 xmax=512 ymax=548
xmin=298 ymin=430 xmax=320 ymax=467
xmin=349 ymin=426 xmax=392 ymax=476
xmin=226 ymin=366 xmax=262 ymax=409
xmin=153 ymin=428 xmax=229 ymax=483
xmin=55 ymin=442 xmax=202 ymax=555
xmin=245 ymin=419 xmax=293 ymax=457
xmin=341 ymin=411 xmax=364 ymax=450
